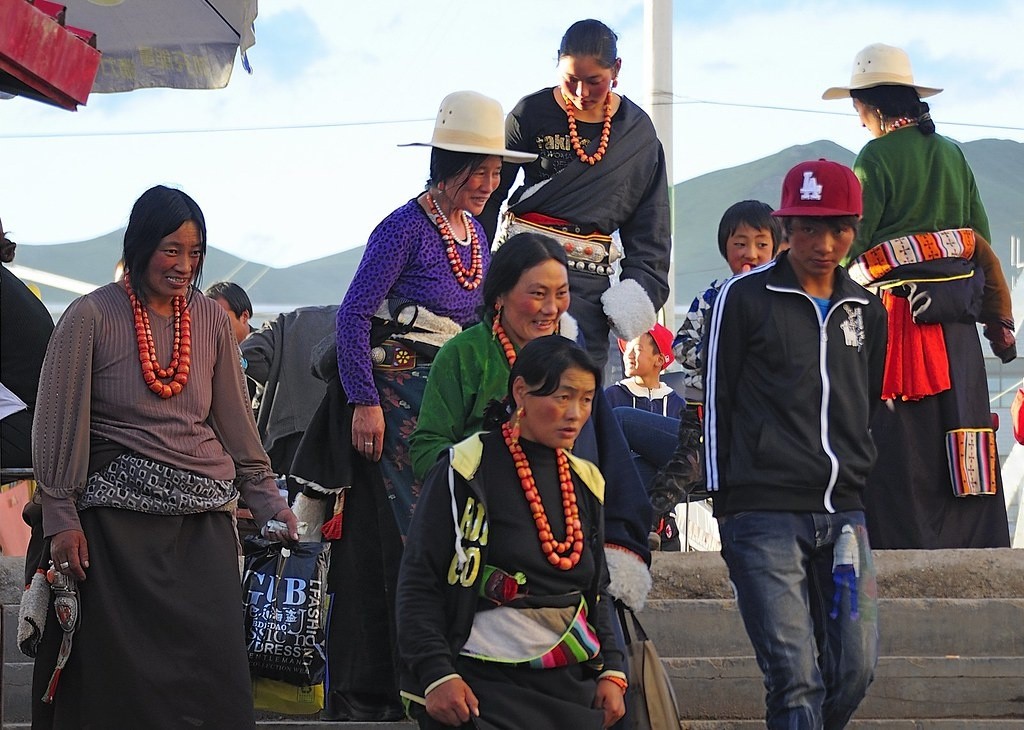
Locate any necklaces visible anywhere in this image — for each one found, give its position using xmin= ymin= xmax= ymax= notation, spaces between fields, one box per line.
xmin=124 ymin=272 xmax=192 ymax=399
xmin=564 ymin=92 xmax=612 ymax=164
xmin=888 ymin=116 xmax=917 ymax=131
xmin=492 ymin=315 xmax=518 ymax=369
xmin=427 ymin=192 xmax=482 ymax=290
xmin=502 ymin=421 xmax=585 ymax=571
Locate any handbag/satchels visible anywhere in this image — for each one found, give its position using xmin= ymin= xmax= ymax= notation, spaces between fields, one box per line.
xmin=609 ymin=595 xmax=683 ymax=730
xmin=251 ymin=594 xmax=331 ymax=715
xmin=240 ymin=532 xmax=325 ymax=687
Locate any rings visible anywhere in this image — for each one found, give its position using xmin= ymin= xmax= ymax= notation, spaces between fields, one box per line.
xmin=365 ymin=442 xmax=372 ymax=446
xmin=60 ymin=562 xmax=68 ymax=569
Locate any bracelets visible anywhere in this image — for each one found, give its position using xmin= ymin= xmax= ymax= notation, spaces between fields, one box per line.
xmin=602 ymin=676 xmax=628 ymax=694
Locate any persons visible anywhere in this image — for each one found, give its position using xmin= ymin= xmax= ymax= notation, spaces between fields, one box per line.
xmin=397 ymin=333 xmax=629 ymax=730
xmin=605 ymin=323 xmax=687 ymax=550
xmin=238 ymin=304 xmax=340 ymax=477
xmin=31 ymin=186 xmax=299 ymax=729
xmin=204 ymin=283 xmax=258 ymax=346
xmin=670 ymin=199 xmax=782 ymax=370
xmin=471 ymin=18 xmax=670 ymax=368
xmin=288 ymin=87 xmax=539 ymax=721
xmin=702 ymin=158 xmax=889 ymax=730
xmin=0 ymin=220 xmax=56 ymax=558
xmin=822 ymin=43 xmax=1017 ymax=549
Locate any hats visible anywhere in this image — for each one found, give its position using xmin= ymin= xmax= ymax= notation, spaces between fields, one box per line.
xmin=397 ymin=90 xmax=539 ymax=163
xmin=618 ymin=322 xmax=675 ymax=371
xmin=821 ymin=44 xmax=943 ymax=99
xmin=770 ymin=158 xmax=863 ymax=217
